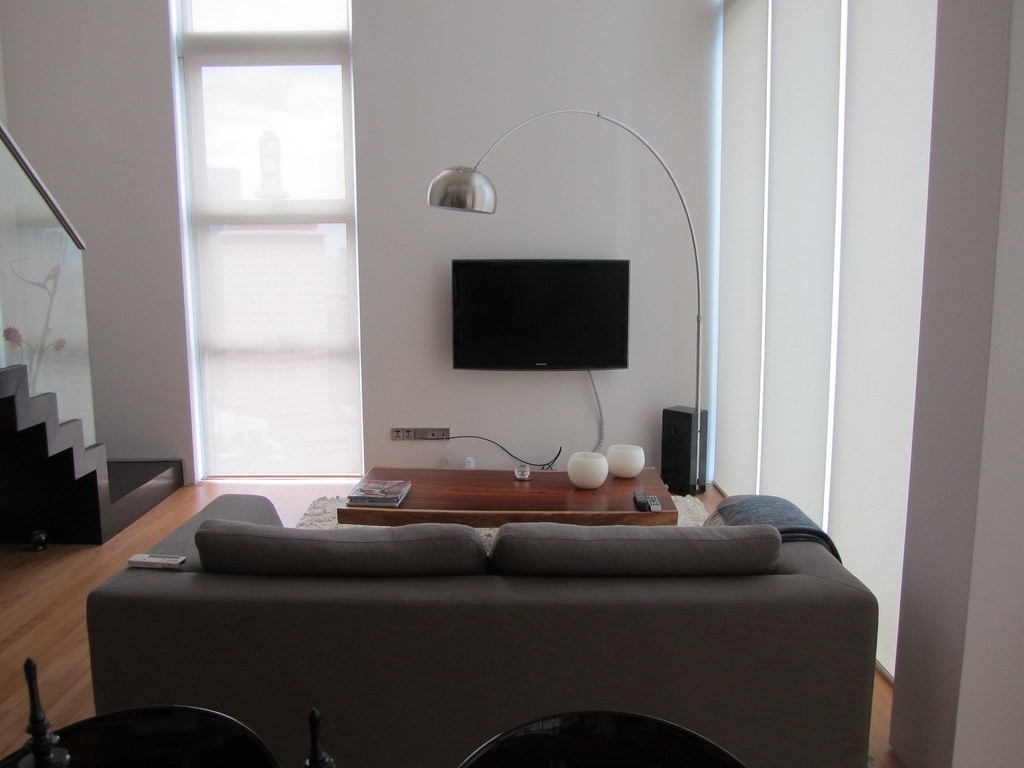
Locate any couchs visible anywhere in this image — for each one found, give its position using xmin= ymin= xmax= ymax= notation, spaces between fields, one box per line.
xmin=86 ymin=494 xmax=878 ymax=768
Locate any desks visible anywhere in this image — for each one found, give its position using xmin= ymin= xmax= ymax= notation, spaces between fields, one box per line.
xmin=337 ymin=466 xmax=678 ymax=527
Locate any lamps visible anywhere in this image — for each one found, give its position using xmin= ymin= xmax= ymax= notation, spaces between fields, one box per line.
xmin=427 ymin=110 xmax=702 ymax=501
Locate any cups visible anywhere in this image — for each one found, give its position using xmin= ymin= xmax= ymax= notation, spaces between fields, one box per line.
xmin=567 ymin=452 xmax=610 ymax=489
xmin=606 ymin=445 xmax=645 ymax=478
xmin=513 ymin=465 xmax=531 ymax=480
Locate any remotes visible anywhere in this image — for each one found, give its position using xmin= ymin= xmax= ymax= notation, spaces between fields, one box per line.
xmin=130 ymin=554 xmax=186 ymax=569
xmin=633 ymin=490 xmax=662 ymax=513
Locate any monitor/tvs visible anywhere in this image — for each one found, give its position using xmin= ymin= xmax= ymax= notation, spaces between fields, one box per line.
xmin=451 ymin=258 xmax=630 ymax=371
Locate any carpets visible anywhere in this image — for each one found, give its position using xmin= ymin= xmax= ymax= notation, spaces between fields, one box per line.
xmin=293 ymin=495 xmax=708 ymax=553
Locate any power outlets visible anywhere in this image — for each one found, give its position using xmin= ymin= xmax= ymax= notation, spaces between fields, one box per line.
xmin=414 ymin=428 xmax=450 ymax=440
xmin=391 ymin=428 xmax=403 ymax=440
xmin=403 ymin=428 xmax=414 ymax=440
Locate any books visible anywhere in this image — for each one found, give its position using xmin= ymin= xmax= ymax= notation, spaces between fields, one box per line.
xmin=346 ymin=478 xmax=412 ymax=508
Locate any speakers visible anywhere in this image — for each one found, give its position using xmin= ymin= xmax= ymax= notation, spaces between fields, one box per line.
xmin=660 ymin=406 xmax=707 ymax=496
